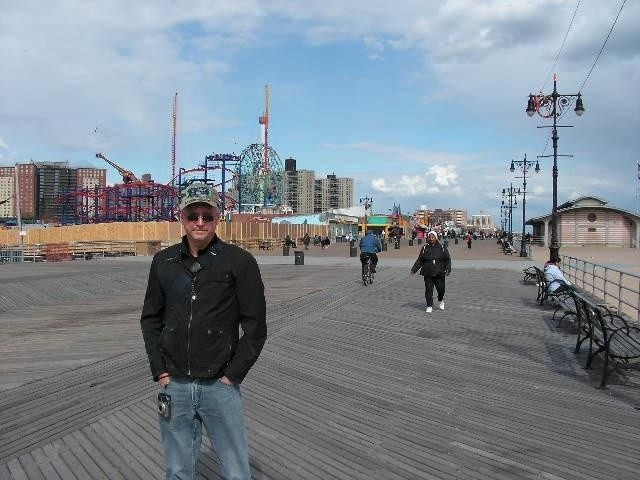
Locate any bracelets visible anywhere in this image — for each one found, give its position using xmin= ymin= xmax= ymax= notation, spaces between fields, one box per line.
xmin=156 ymin=375 xmax=172 ymax=381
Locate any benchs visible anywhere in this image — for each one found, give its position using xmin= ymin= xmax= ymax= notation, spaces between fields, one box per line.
xmin=522 ymin=265 xmax=610 ymax=328
xmin=571 ymin=290 xmax=640 ymax=390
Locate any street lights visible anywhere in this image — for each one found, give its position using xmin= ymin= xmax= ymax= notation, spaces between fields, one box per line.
xmin=359 ymin=194 xmax=373 ymax=235
xmin=510 ymin=155 xmax=541 ymax=257
xmin=526 ymin=74 xmax=586 ymax=263
xmin=501 ymin=183 xmax=520 ymax=236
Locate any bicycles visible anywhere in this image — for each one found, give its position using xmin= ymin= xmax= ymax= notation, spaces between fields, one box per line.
xmin=362 ymin=250 xmax=381 ymax=286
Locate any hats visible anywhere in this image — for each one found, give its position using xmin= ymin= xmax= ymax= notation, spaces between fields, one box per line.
xmin=180 ymin=183 xmax=219 ymax=209
xmin=366 ymin=230 xmax=373 ymax=233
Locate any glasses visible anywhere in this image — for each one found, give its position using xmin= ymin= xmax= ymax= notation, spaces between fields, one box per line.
xmin=186 ymin=214 xmax=214 ymax=222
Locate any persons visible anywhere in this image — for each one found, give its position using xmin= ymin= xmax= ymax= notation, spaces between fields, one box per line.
xmin=359 ymin=230 xmax=382 ymax=280
xmin=285 ymin=231 xmax=517 ymax=253
xmin=543 ymin=257 xmax=575 ymax=293
xmin=409 ymin=231 xmax=451 ymax=313
xmin=141 ymin=183 xmax=267 ymax=480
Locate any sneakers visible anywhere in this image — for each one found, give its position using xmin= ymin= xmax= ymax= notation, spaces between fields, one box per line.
xmin=439 ymin=300 xmax=445 ymax=310
xmin=425 ymin=306 xmax=434 ymax=314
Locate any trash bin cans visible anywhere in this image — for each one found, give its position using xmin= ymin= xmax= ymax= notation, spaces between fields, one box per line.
xmin=336 ymin=235 xmax=346 ymax=242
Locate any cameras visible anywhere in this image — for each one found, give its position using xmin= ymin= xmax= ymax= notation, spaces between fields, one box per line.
xmin=157 ymin=393 xmax=171 ymax=419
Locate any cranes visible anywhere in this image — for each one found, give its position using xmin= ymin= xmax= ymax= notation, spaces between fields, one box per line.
xmin=258 ymin=83 xmax=271 ymax=171
xmin=94 ymin=152 xmax=139 ymax=182
xmin=170 ymin=90 xmax=179 ymax=185
